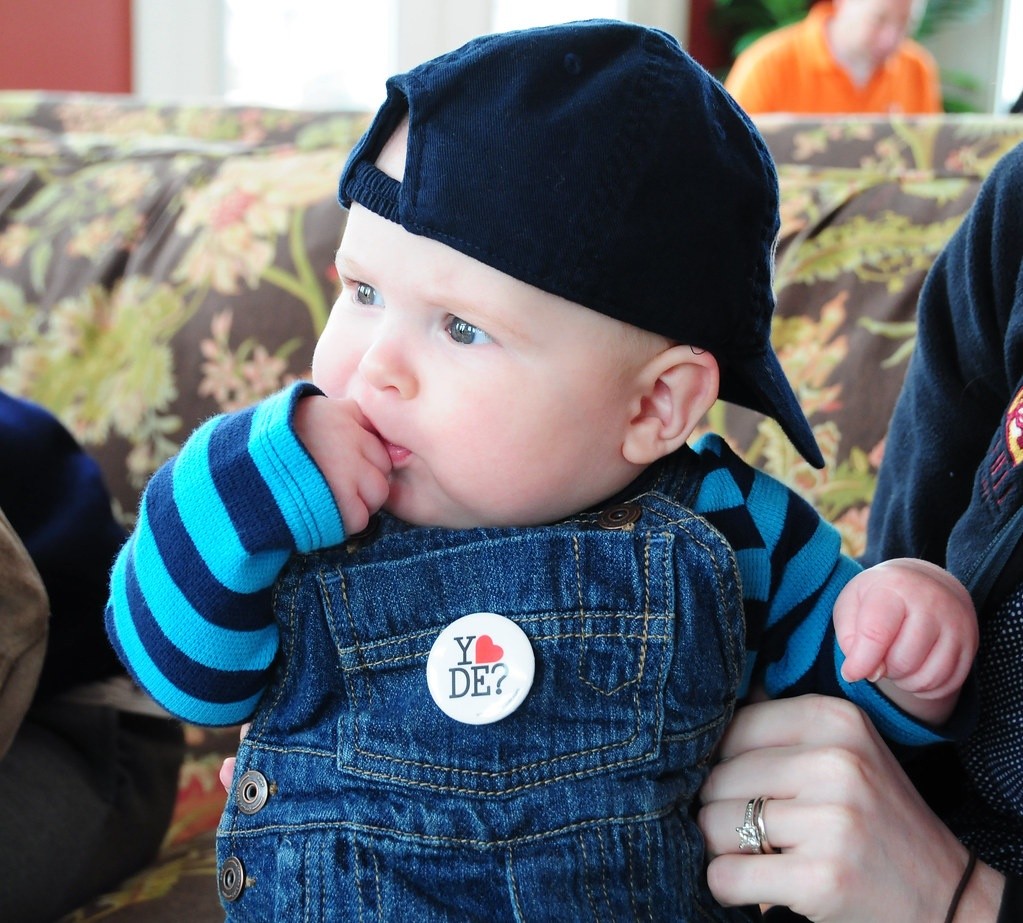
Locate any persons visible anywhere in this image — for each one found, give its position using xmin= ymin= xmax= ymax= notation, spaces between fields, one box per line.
xmin=724 ymin=0 xmax=944 ymax=115
xmin=0 ymin=19 xmax=1023 ymax=923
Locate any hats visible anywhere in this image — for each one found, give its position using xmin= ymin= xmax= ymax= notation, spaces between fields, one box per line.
xmin=336 ymin=18 xmax=827 ymax=469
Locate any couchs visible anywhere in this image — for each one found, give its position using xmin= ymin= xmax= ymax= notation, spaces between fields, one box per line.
xmin=0 ymin=87 xmax=1023 ymax=923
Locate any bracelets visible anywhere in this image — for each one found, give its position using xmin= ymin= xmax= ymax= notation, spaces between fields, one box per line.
xmin=943 ymin=849 xmax=976 ymax=923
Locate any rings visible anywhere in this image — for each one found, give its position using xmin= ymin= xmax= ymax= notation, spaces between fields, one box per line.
xmin=736 ymin=797 xmax=762 ymax=854
xmin=755 ymin=796 xmax=778 ymax=854
